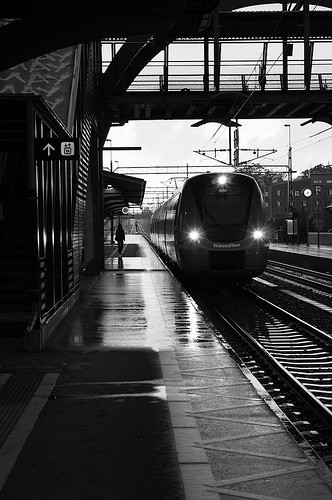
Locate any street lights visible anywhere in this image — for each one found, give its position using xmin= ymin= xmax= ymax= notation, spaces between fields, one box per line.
xmin=282 ymin=124 xmax=292 ymax=171
xmin=104 ymin=139 xmax=113 ymax=172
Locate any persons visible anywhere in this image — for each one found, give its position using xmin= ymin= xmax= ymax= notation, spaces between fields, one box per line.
xmin=115 ymin=224 xmax=125 ymax=255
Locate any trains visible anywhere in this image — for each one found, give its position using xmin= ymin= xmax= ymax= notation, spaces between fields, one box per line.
xmin=150 ymin=172 xmax=270 ymax=286
